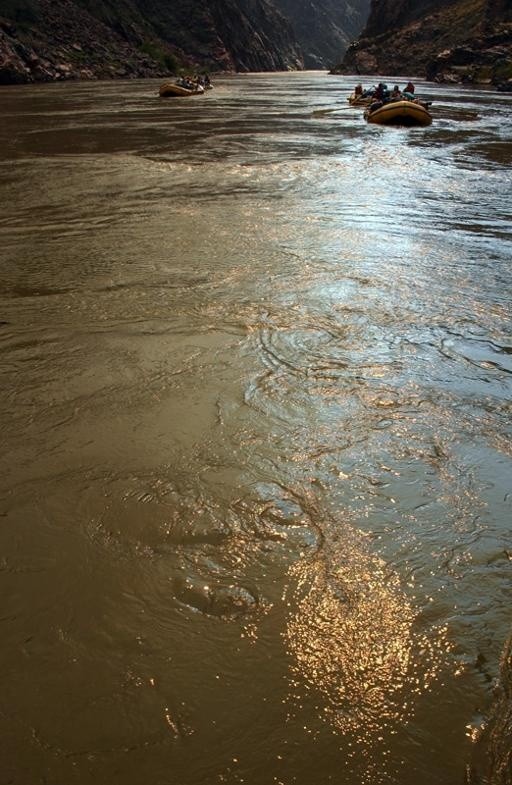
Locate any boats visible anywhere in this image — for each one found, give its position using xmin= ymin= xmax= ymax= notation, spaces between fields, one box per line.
xmin=361 ymin=98 xmax=436 ymax=126
xmin=349 ymin=92 xmax=382 ymax=105
xmin=158 ymin=80 xmax=213 ymax=97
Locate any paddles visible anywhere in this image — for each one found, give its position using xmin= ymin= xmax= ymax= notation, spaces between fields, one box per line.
xmin=350 ymin=84 xmax=374 ymax=104
xmin=428 ymin=105 xmax=468 ymax=113
xmin=312 ymin=105 xmax=369 ymax=113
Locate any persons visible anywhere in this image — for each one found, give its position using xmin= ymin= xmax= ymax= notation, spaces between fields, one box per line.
xmin=354 ymin=81 xmax=419 ymax=111
xmin=175 ymin=71 xmax=211 ymax=90
